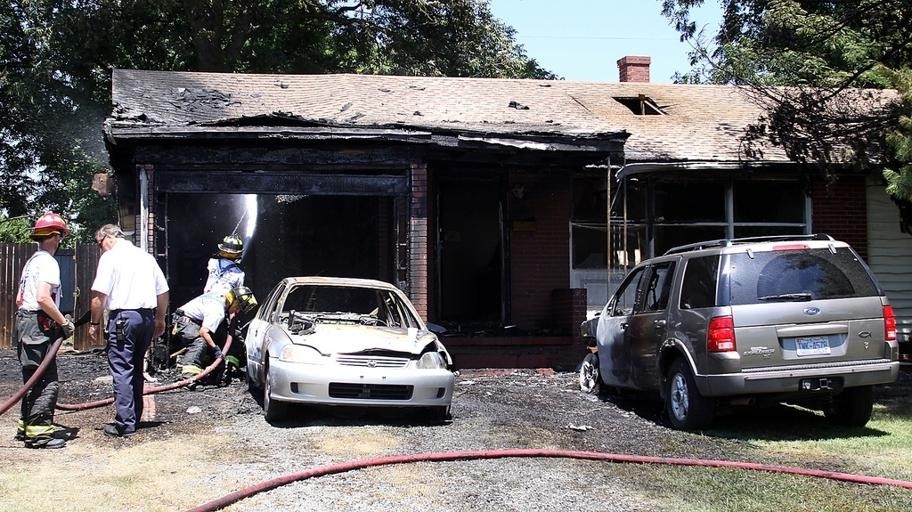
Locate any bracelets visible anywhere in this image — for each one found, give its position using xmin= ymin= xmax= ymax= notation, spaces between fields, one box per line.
xmin=90 ymin=321 xmax=100 ymax=326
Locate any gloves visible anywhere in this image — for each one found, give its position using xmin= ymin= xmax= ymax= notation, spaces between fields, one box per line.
xmin=60 ymin=319 xmax=76 ymax=339
xmin=212 ymin=344 xmax=226 ymax=361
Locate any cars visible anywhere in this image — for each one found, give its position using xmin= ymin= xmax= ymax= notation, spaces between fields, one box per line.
xmin=244 ymin=276 xmax=455 ymax=422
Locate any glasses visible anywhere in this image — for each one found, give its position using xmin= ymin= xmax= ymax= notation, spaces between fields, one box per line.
xmin=98 ymin=236 xmax=107 ymax=250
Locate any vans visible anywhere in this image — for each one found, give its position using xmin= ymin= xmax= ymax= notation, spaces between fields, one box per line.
xmin=579 ymin=233 xmax=891 ymax=430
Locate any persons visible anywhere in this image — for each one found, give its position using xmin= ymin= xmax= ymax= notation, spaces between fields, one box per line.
xmin=203 ymin=234 xmax=246 ymax=293
xmin=172 ymin=286 xmax=257 ymax=378
xmin=15 ymin=213 xmax=77 ymax=449
xmin=89 ymin=224 xmax=172 ymax=435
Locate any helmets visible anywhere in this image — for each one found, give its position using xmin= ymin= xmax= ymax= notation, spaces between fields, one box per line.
xmin=217 ymin=236 xmax=244 ymax=259
xmin=30 ymin=213 xmax=69 ymax=241
xmin=228 ymin=284 xmax=259 ymax=315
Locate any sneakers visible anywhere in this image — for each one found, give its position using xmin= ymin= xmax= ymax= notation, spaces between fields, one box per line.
xmin=182 ymin=364 xmax=211 ymax=379
xmin=102 ymin=424 xmax=137 ymax=440
xmin=25 ymin=437 xmax=65 ymax=450
xmin=15 ymin=431 xmax=28 ymax=441
